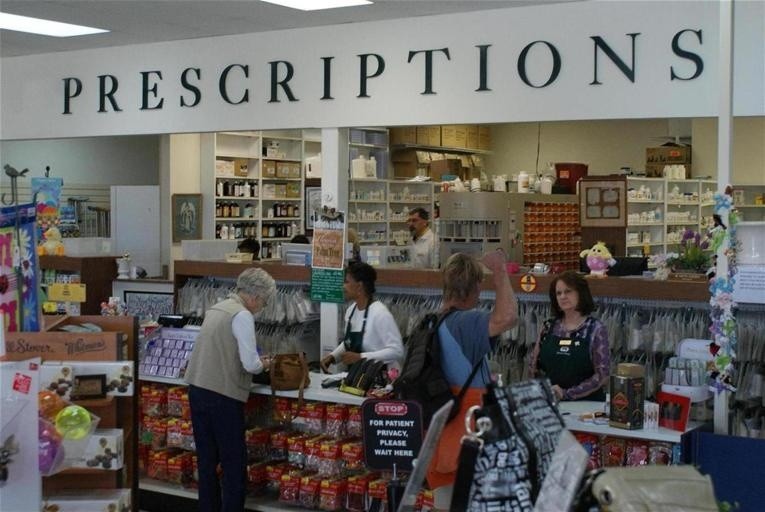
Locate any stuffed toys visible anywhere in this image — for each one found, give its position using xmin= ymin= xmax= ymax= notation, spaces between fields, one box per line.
xmin=37 ymin=227 xmax=65 ymax=257
xmin=579 ymin=241 xmax=617 ymax=277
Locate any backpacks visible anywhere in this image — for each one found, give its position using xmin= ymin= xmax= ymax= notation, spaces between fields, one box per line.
xmin=393 ymin=308 xmax=484 ymax=429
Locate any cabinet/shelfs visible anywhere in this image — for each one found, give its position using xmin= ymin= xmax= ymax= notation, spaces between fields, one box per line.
xmin=137 ymin=370 xmax=713 ymax=512
xmin=0 ymin=331 xmax=123 ymax=491
xmin=730 ymin=182 xmax=765 ymax=261
xmin=202 ymin=128 xmax=321 ymax=256
xmin=578 ymin=172 xmax=718 ymax=270
xmin=439 ymin=192 xmax=581 ymax=274
xmin=345 ymin=127 xmax=485 ymax=269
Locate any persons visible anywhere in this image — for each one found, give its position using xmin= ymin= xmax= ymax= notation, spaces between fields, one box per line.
xmin=410 ymin=248 xmax=519 ymax=512
xmin=406 ymin=207 xmax=441 ymax=269
xmin=320 ymin=261 xmax=405 ymax=373
xmin=184 ymin=267 xmax=274 ymax=512
xmin=237 ymin=238 xmax=261 ymax=261
xmin=526 ymin=271 xmax=613 ymax=404
xmin=290 ymin=235 xmax=310 ymax=244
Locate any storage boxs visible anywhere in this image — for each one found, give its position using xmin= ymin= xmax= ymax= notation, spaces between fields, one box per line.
xmin=644 ymin=141 xmax=691 ymax=164
xmin=645 ymin=162 xmax=690 ymax=177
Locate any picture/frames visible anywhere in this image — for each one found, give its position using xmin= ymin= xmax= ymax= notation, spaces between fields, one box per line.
xmin=171 ymin=193 xmax=202 ymax=242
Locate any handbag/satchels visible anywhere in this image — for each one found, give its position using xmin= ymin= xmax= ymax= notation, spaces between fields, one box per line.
xmin=269 ymin=352 xmax=310 ymax=391
xmin=585 ymin=462 xmax=721 ymax=512
xmin=451 ymin=377 xmax=589 ymax=512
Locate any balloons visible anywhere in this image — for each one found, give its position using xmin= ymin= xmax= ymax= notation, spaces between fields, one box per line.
xmin=55 ymin=404 xmax=91 ymax=441
xmin=38 ymin=434 xmax=66 ymax=476
xmin=40 ymin=420 xmax=51 ymax=439
xmin=38 ymin=390 xmax=62 ymax=423
